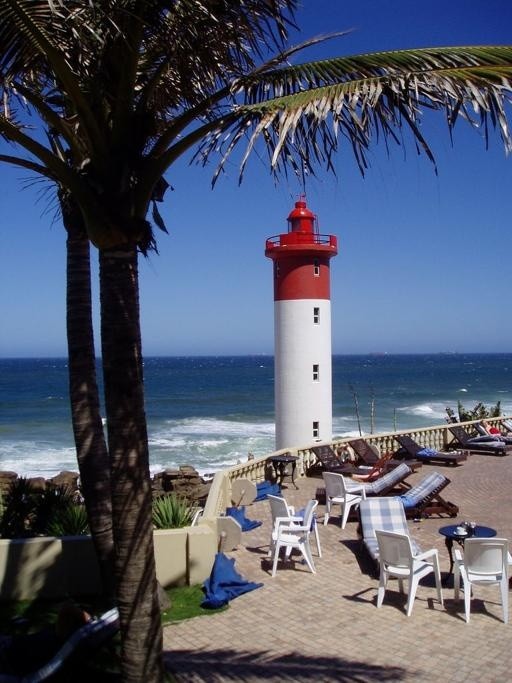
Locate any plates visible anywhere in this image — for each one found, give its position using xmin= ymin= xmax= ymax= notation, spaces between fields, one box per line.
xmin=453 ymin=532 xmax=468 ymax=536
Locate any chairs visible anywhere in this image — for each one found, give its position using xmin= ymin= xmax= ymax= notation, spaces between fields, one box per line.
xmin=451 ymin=536 xmax=511 ymax=625
xmin=375 ymin=529 xmax=445 ymax=618
xmin=265 ymin=492 xmax=322 ymax=578
xmin=322 ymin=471 xmax=367 ymax=530
xmin=0 ymin=606 xmax=122 ymax=683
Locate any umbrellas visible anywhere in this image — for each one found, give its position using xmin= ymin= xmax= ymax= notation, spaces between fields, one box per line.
xmin=202 ymin=531 xmax=263 ymax=609
xmin=255 ymin=474 xmax=285 ymax=502
xmin=221 ymin=490 xmax=263 ymax=532
xmin=294 ymin=508 xmax=319 ymax=534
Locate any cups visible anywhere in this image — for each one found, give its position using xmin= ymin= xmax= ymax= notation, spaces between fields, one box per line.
xmin=456 ymin=527 xmax=466 ymax=534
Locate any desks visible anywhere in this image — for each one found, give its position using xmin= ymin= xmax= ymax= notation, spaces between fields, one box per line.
xmin=265 ymin=455 xmax=301 ymax=492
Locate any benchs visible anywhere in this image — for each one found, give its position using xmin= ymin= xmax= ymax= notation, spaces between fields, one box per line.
xmin=439 ymin=522 xmax=497 ymax=582
xmin=441 ymin=422 xmax=512 ymax=455
xmin=358 ymin=496 xmax=427 ymax=566
xmin=310 ymin=435 xmax=467 ymax=518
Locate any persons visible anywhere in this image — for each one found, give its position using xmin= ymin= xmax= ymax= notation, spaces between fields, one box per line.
xmin=0 ymin=603 xmax=95 ymax=678
xmin=480 ymin=419 xmax=512 ymax=436
